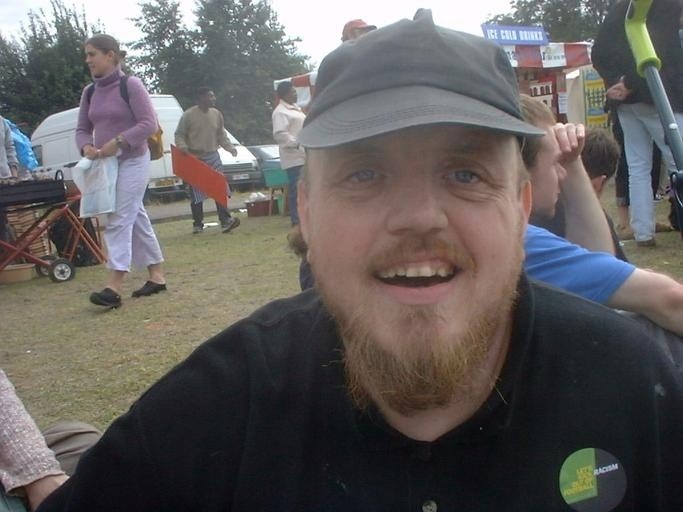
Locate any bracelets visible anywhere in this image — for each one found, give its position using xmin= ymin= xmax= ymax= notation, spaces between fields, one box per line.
xmin=115 ymin=135 xmax=124 ymax=150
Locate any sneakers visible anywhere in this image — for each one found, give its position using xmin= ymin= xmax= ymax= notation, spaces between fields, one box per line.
xmin=222 ymin=217 xmax=240 ymax=233
xmin=193 ymin=226 xmax=202 ymax=233
xmin=618 ymin=222 xmax=673 ymax=247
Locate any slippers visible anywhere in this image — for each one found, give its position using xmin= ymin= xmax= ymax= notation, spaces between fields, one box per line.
xmin=90 ymin=287 xmax=120 ymax=306
xmin=132 ymin=280 xmax=166 ymax=296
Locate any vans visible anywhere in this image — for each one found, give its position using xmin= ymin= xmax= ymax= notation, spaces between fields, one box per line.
xmin=217 ymin=126 xmax=262 ymax=192
xmin=25 ymin=92 xmax=187 ymax=204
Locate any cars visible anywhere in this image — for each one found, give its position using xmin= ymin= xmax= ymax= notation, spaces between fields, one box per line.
xmin=246 ymin=142 xmax=291 ymax=187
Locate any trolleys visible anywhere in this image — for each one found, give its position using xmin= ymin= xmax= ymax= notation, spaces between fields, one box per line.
xmin=0 ymin=168 xmax=75 ymax=284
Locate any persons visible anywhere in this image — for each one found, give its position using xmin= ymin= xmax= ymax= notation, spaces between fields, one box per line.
xmin=603 ymin=100 xmax=675 ymax=240
xmin=590 ymin=0 xmax=682 ymax=249
xmin=517 ymin=90 xmax=682 ymax=380
xmin=33 ymin=8 xmax=682 ymax=512
xmin=655 ymin=153 xmax=670 ymax=202
xmin=527 ymin=124 xmax=629 ymax=264
xmin=270 ymin=81 xmax=307 ymax=225
xmin=73 ymin=34 xmax=169 ymax=309
xmin=0 ymin=114 xmax=20 ymax=254
xmin=342 ymin=19 xmax=381 ymax=43
xmin=0 ymin=364 xmax=105 ymax=511
xmin=173 ymin=87 xmax=241 ymax=235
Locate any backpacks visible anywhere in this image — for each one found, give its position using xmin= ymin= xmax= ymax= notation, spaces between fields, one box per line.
xmin=86 ymin=75 xmax=163 ymax=160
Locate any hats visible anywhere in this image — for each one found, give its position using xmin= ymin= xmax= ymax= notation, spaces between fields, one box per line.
xmin=297 ymin=7 xmax=547 ymax=148
xmin=340 ymin=19 xmax=377 ymax=41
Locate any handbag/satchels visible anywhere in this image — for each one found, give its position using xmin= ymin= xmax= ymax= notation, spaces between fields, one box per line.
xmin=71 ymin=150 xmax=121 ymax=218
